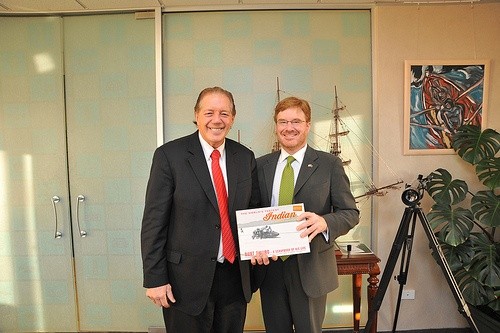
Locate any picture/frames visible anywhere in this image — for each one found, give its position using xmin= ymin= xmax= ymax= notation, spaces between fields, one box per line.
xmin=403 ymin=59 xmax=490 ymax=156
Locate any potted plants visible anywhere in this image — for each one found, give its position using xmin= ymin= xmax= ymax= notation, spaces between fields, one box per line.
xmin=426 ymin=124 xmax=500 ymax=333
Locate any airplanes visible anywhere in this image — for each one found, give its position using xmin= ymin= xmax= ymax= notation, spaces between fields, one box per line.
xmin=252 ymin=225 xmax=279 ymax=239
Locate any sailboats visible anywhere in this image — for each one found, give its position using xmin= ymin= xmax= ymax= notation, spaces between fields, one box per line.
xmin=238 ymin=77 xmax=404 ymax=250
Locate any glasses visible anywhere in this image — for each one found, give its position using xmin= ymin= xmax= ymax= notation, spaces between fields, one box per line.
xmin=278 ymin=118 xmax=305 ymax=125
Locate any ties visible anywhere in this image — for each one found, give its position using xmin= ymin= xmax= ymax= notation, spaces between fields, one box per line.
xmin=210 ymin=149 xmax=236 ymax=263
xmin=278 ymin=155 xmax=294 ymax=260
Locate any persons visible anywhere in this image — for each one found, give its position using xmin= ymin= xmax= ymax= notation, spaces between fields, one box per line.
xmin=140 ymin=86 xmax=278 ymax=333
xmin=255 ymin=96 xmax=360 ymax=333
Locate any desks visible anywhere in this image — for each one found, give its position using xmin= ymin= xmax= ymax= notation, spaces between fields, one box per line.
xmin=334 ymin=240 xmax=381 ymax=333
